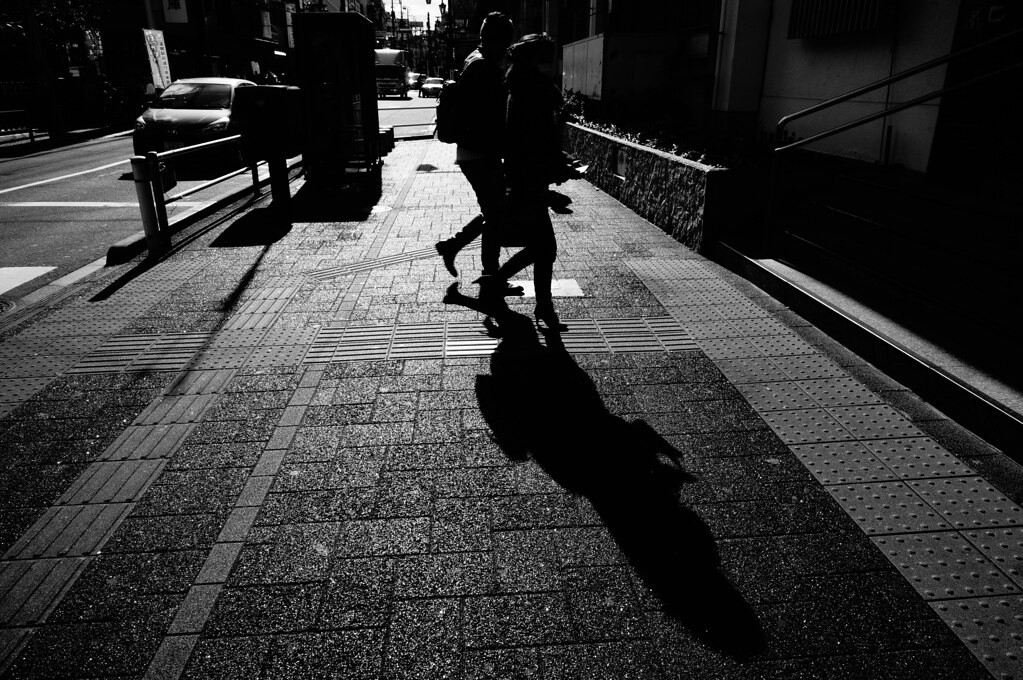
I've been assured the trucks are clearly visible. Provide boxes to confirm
[373,48,410,98]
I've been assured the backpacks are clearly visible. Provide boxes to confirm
[437,56,493,143]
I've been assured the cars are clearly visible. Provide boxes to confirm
[131,77,258,158]
[421,77,444,98]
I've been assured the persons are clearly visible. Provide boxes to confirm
[434,11,588,332]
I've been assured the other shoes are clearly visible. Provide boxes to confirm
[503,281,524,294]
[435,238,460,278]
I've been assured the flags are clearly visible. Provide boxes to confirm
[143,29,171,89]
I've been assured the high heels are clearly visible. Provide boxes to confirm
[533,303,568,329]
[472,275,500,317]
